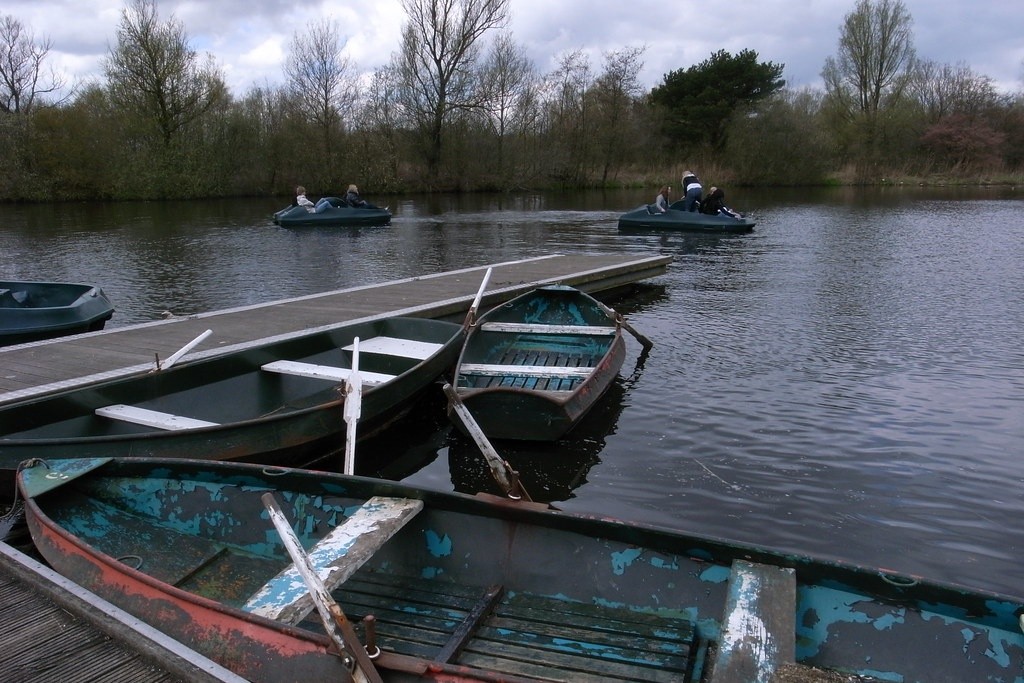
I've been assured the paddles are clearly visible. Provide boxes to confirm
[593,299,656,352]
[256,487,386,683]
[147,326,215,376]
[436,378,539,507]
[342,334,365,482]
[459,264,495,343]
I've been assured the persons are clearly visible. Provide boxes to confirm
[295,185,340,213]
[681,169,703,211]
[655,185,671,213]
[697,187,742,221]
[346,183,389,210]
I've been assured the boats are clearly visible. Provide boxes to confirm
[618,203,758,234]
[0,276,117,346]
[272,196,394,227]
[445,282,628,441]
[14,453,1024,683]
[0,317,470,483]
